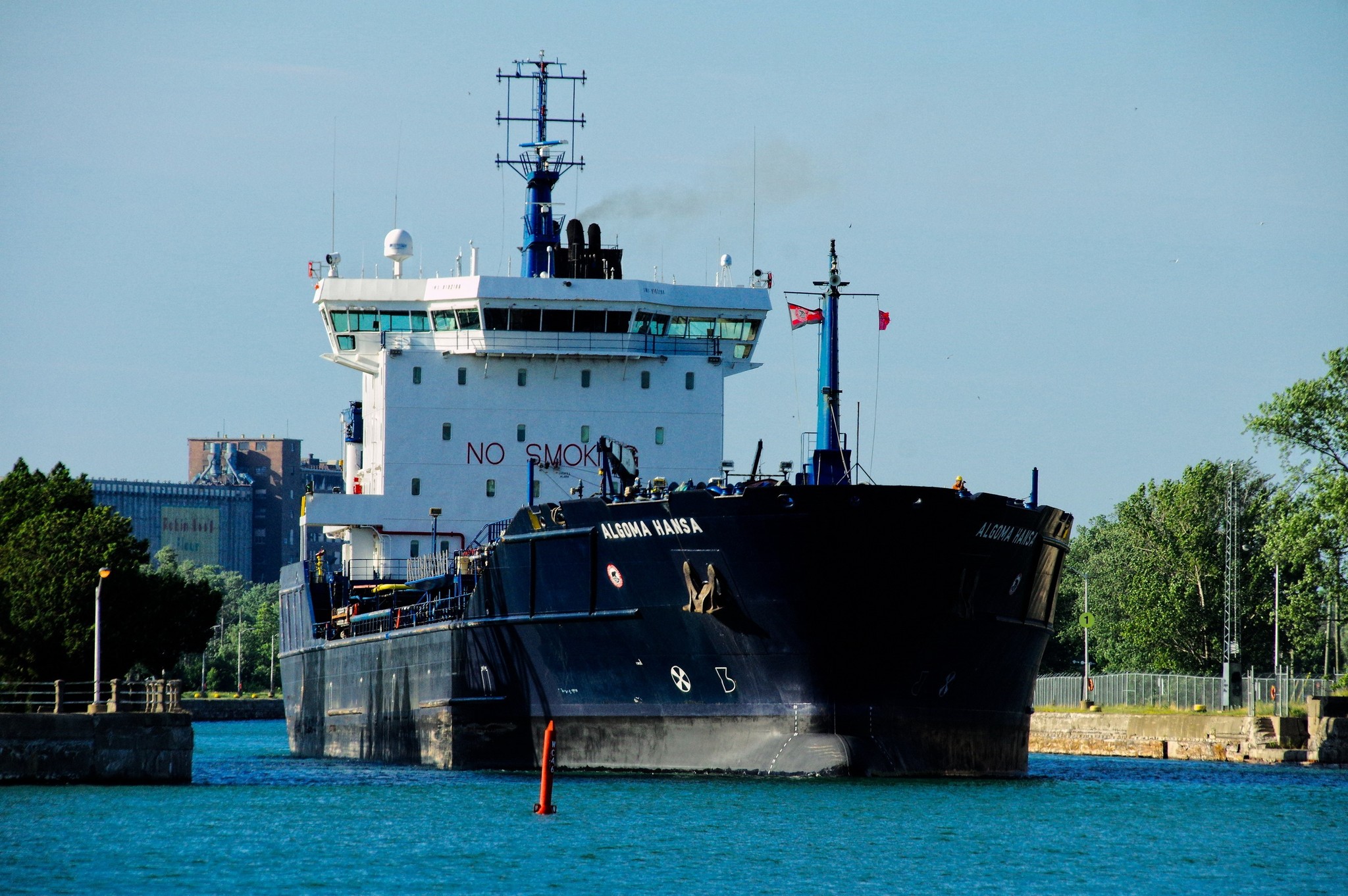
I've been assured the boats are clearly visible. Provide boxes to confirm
[276,47,1076,779]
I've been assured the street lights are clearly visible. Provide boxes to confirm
[269,633,279,697]
[1063,566,1089,701]
[428,507,443,577]
[237,627,257,696]
[201,624,221,693]
[1241,544,1279,674]
[94,567,111,705]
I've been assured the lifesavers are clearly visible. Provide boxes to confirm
[396,609,401,629]
[1088,679,1094,690]
[1271,685,1276,701]
[355,603,359,615]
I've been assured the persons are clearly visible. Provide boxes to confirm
[952,476,966,489]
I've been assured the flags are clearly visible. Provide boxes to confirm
[787,301,825,330]
[879,310,890,330]
